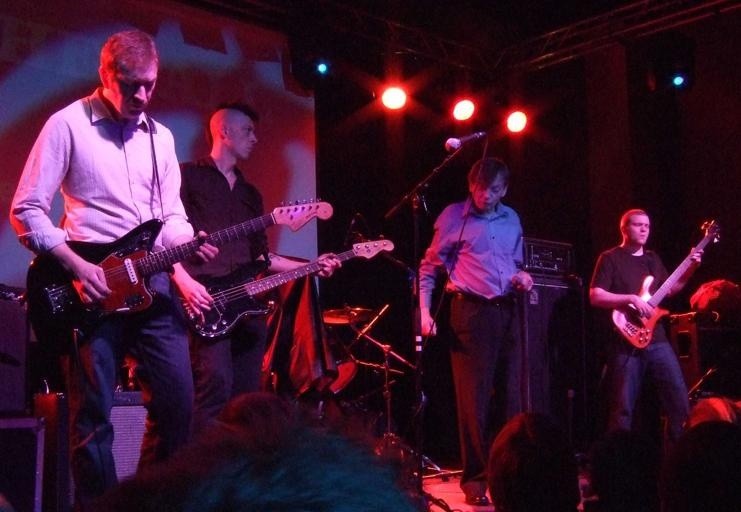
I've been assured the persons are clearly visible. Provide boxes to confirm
[588,208,705,456]
[413,157,534,506]
[179,101,342,443]
[486,396,741,512]
[116,393,421,512]
[9,29,221,497]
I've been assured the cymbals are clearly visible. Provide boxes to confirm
[324,308,375,323]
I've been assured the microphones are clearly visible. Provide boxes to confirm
[444,131,488,151]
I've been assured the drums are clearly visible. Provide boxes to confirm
[324,328,357,395]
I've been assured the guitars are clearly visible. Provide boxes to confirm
[612,220,720,348]
[177,234,393,343]
[26,197,334,356]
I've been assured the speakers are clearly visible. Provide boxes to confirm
[668,313,741,397]
[494,271,586,448]
